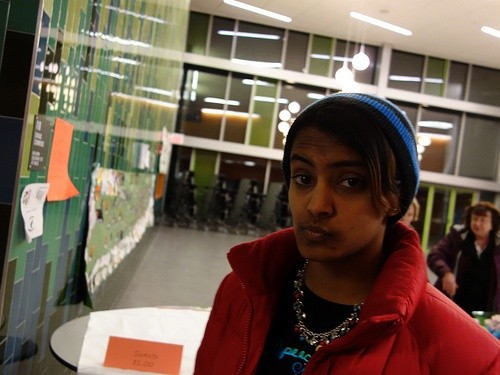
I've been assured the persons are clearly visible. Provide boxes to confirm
[399,193,421,227]
[426,202,500,317]
[192,92,500,375]
[167,169,291,236]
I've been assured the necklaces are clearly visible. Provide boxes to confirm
[293,256,369,347]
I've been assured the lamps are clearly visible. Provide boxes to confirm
[480,25,500,40]
[349,11,413,38]
[224,0,292,23]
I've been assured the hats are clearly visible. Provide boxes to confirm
[282,92,420,224]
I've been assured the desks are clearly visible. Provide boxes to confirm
[50,306,211,375]
